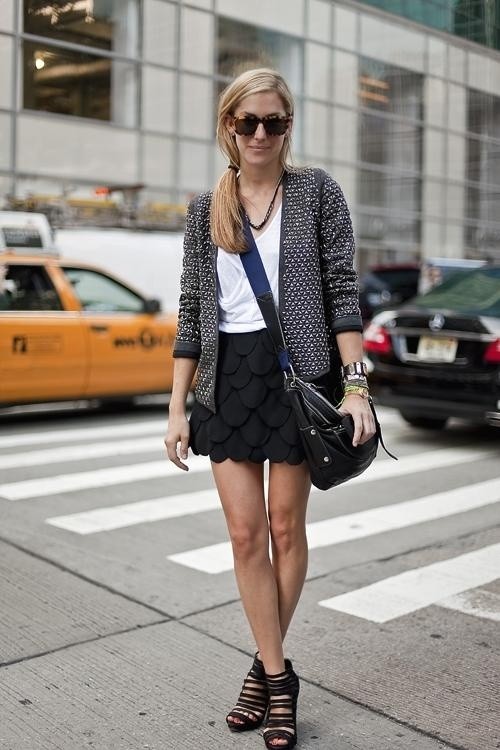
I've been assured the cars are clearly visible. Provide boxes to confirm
[0,251,197,406]
[359,255,499,435]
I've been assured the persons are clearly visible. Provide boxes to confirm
[164,66,378,749]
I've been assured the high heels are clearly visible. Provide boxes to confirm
[226,651,299,749]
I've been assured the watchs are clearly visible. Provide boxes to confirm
[343,385,369,398]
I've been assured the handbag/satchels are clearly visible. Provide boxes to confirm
[286,365,398,490]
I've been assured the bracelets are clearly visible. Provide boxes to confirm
[340,362,370,389]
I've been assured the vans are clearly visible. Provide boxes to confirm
[1,209,57,259]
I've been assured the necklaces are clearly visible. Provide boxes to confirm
[237,168,288,230]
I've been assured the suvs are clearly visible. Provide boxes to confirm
[355,258,491,329]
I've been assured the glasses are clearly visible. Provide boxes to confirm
[230,112,288,136]
